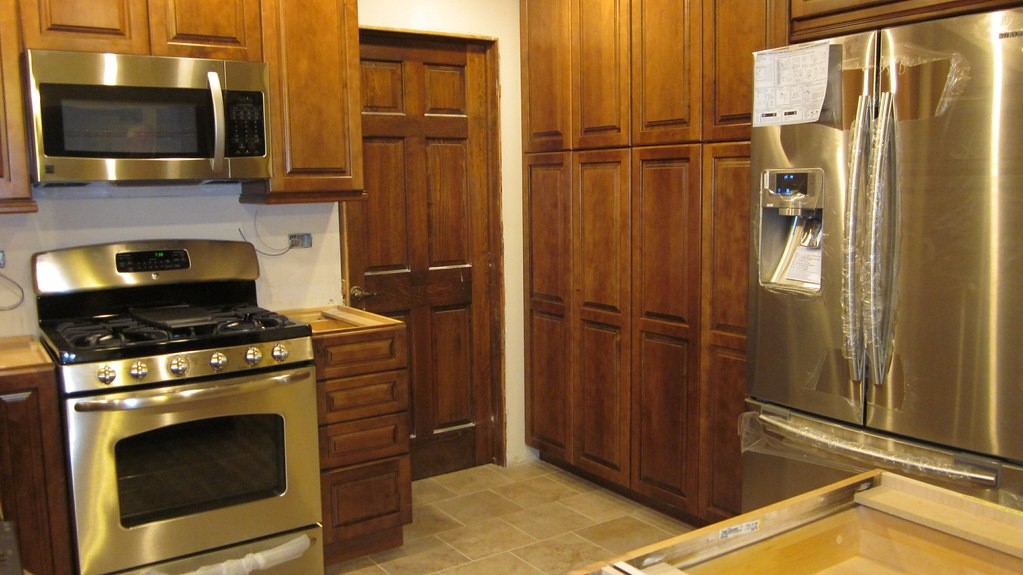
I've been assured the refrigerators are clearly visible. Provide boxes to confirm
[742,5,1023,534]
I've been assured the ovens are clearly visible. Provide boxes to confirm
[59,365,332,575]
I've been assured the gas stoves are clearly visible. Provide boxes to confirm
[29,238,315,393]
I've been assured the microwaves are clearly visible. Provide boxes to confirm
[21,45,273,186]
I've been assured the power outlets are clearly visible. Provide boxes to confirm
[288,233,313,249]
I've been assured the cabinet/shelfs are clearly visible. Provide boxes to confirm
[238,0,369,204]
[520,0,791,530]
[0,365,74,575]
[17,0,263,62]
[0,0,39,214]
[312,323,415,567]
